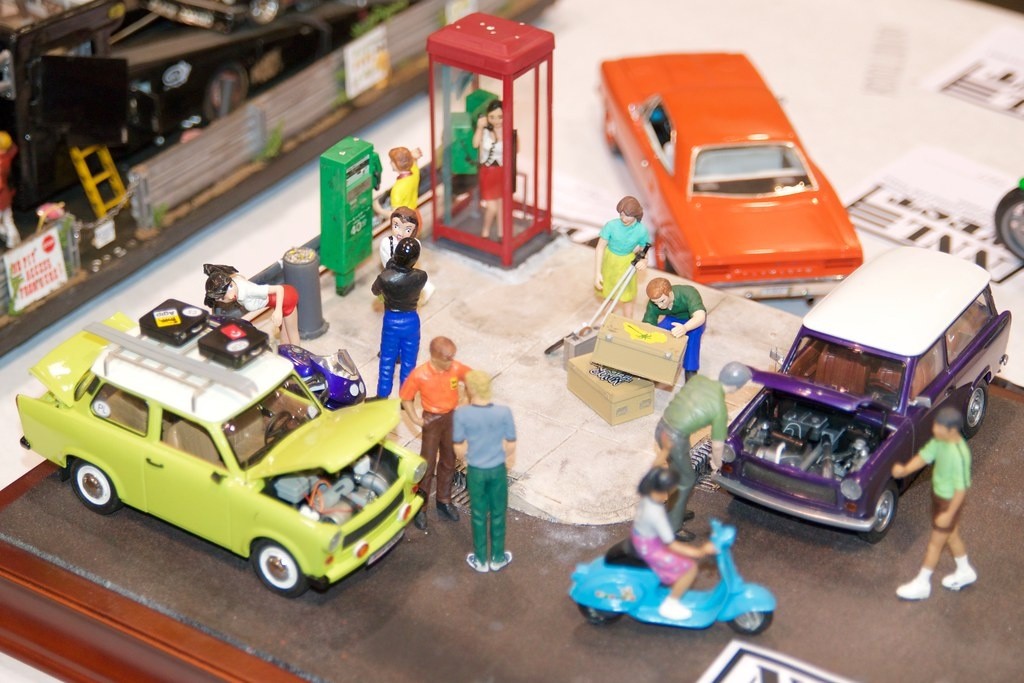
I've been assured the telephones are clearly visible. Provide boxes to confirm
[372,170,382,190]
[477,112,494,132]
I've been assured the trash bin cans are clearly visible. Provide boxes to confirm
[283,249,331,338]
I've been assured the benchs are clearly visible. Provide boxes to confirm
[170,403,267,464]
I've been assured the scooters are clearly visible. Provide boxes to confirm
[568,519,776,637]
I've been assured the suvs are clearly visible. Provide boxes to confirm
[706,242,1012,544]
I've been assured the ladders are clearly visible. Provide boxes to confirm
[71,138,130,222]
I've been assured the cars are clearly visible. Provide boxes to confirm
[597,52,864,306]
[14,309,429,599]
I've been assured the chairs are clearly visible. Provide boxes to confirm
[877,361,930,398]
[814,344,871,397]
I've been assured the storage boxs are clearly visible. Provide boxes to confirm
[591,312,689,386]
[566,352,655,427]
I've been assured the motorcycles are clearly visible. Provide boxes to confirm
[278,342,366,412]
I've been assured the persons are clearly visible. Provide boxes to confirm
[373,144,422,240]
[451,368,520,572]
[891,408,979,598]
[378,205,418,268]
[592,196,651,319]
[641,276,708,387]
[399,336,476,529]
[472,99,506,240]
[202,264,302,348]
[631,431,719,623]
[363,238,427,411]
[656,361,753,543]
